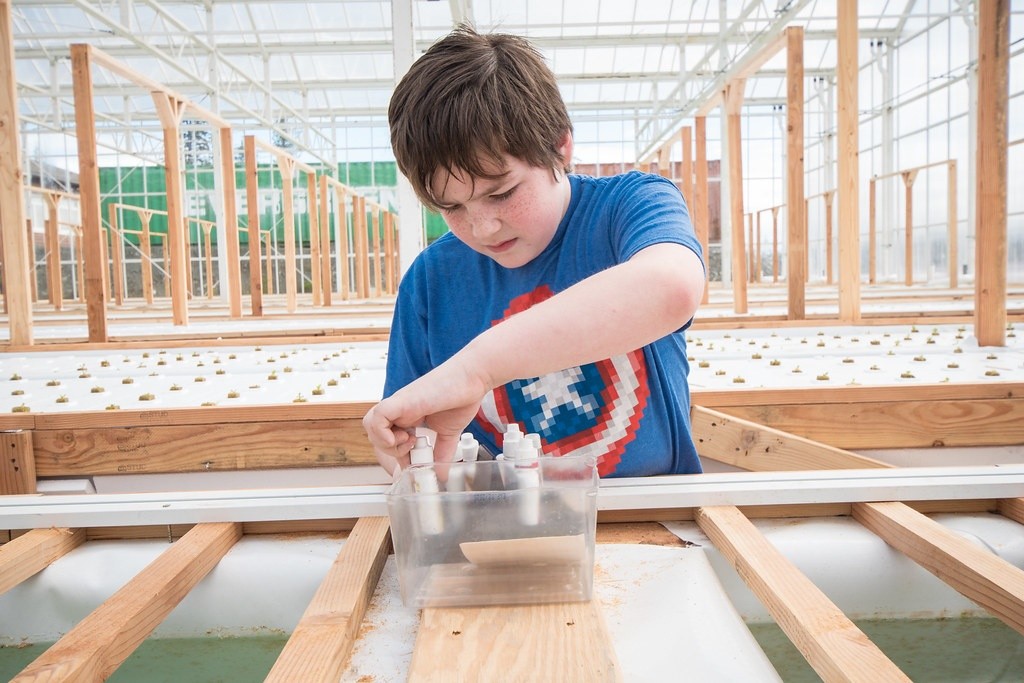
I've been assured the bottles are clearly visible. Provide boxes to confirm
[409,425,544,538]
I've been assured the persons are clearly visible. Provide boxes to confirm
[363,20,707,491]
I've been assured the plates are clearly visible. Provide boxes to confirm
[458,534,586,568]
